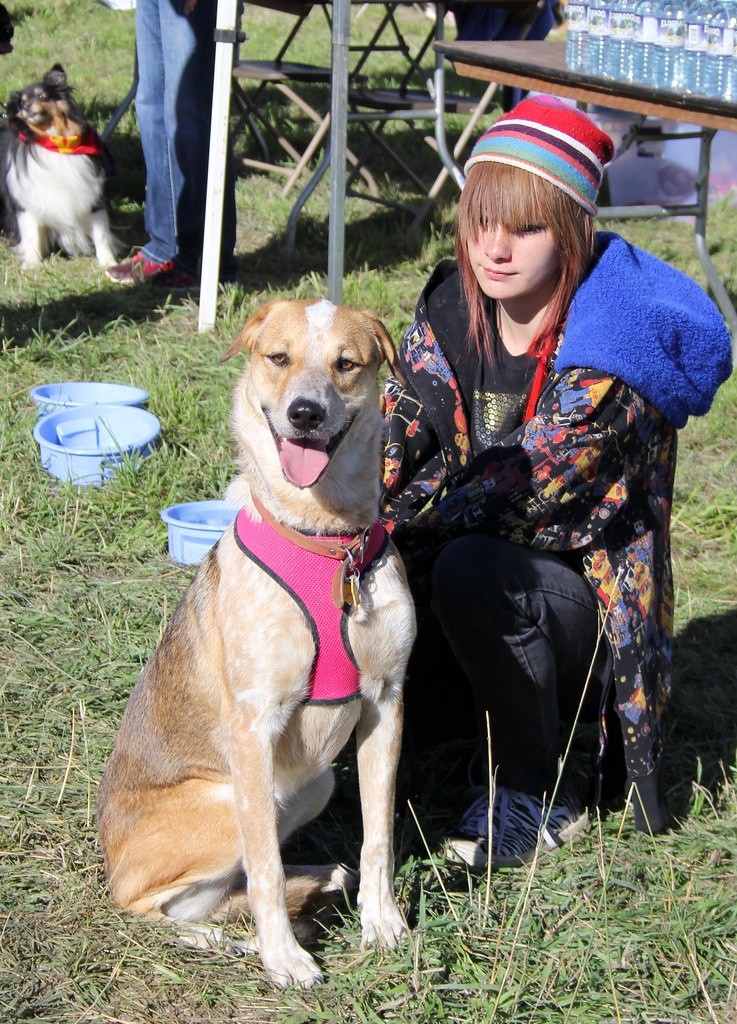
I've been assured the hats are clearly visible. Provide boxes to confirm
[462,92,614,217]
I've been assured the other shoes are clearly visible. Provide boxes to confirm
[105,252,198,291]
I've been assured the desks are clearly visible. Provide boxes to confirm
[431,37,737,340]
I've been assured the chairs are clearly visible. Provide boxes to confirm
[100,0,545,253]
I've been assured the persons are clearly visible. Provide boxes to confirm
[453,0,567,98]
[381,95,732,867]
[105,1,247,293]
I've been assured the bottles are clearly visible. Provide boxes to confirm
[563,0,737,105]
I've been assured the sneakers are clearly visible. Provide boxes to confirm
[444,775,590,872]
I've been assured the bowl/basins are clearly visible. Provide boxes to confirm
[161,500,242,566]
[33,405,160,488]
[30,380,149,419]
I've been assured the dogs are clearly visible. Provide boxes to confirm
[95,299,420,996]
[1,61,125,276]
